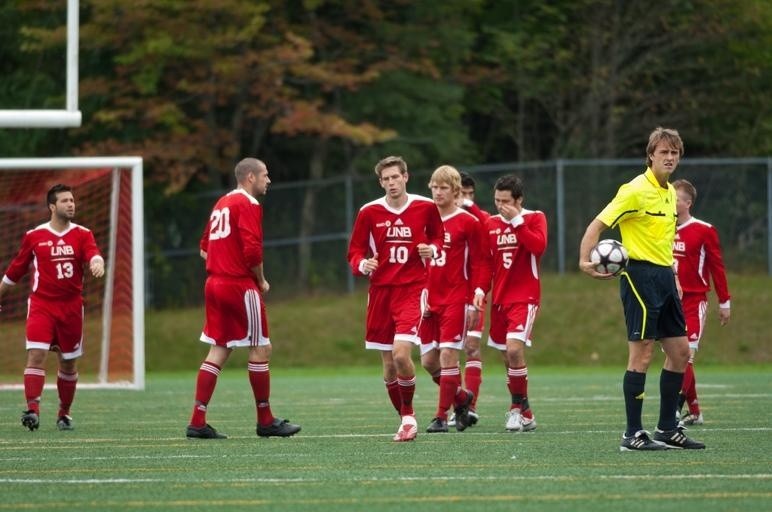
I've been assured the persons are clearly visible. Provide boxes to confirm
[472,177,548,433]
[458,172,492,427]
[671,179,732,426]
[346,154,444,442]
[0,184,105,430]
[186,153,301,437]
[580,128,711,452]
[420,165,483,432]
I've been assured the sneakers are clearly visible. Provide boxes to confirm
[678,412,704,425]
[256,418,300,437]
[652,426,705,449]
[57,413,73,431]
[619,430,666,452]
[448,389,479,431]
[20,410,40,431]
[506,409,522,431]
[518,414,537,432]
[391,411,450,442]
[186,424,227,440]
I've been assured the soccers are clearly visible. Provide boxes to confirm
[588,238,629,278]
